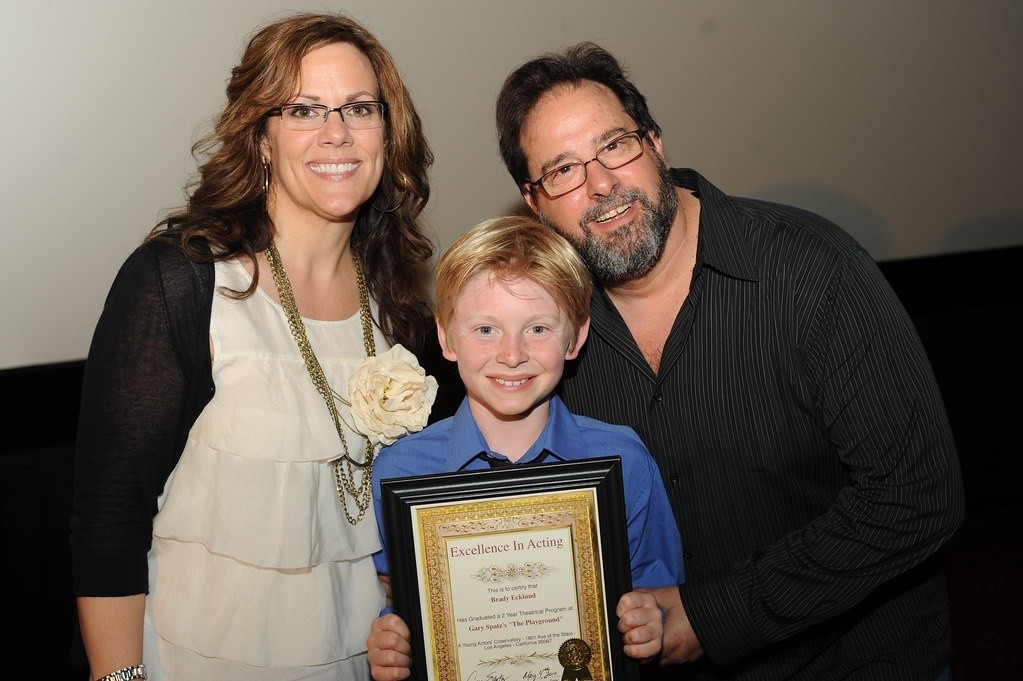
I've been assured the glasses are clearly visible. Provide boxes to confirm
[524,128,644,200]
[265,100,388,130]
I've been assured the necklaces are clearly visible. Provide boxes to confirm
[261,217,378,527]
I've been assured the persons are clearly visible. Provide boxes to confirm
[65,11,472,681]
[492,40,971,681]
[367,211,690,681]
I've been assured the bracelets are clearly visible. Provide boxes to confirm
[94,663,148,681]
